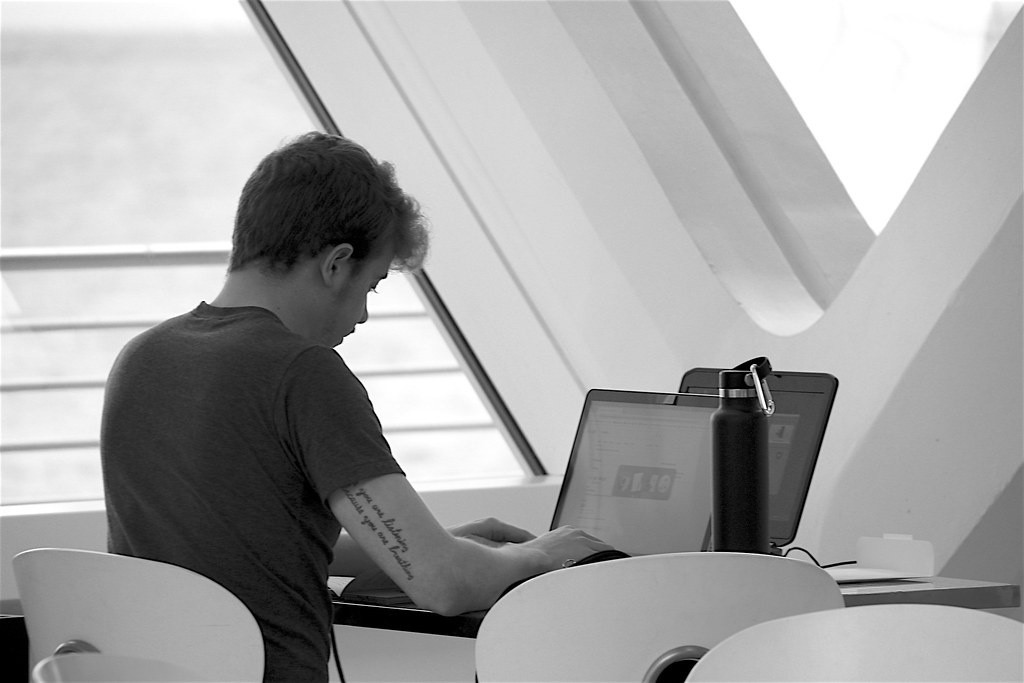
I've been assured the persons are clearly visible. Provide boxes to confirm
[99,132,621,683]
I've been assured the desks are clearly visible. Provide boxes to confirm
[323,561,1020,683]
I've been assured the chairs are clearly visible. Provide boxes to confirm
[684,604,1024,683]
[475,551,844,683]
[12,548,265,683]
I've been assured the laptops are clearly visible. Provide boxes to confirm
[549,368,836,558]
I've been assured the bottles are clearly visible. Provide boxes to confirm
[711,358,774,553]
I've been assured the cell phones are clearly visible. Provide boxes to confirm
[341,588,411,604]
